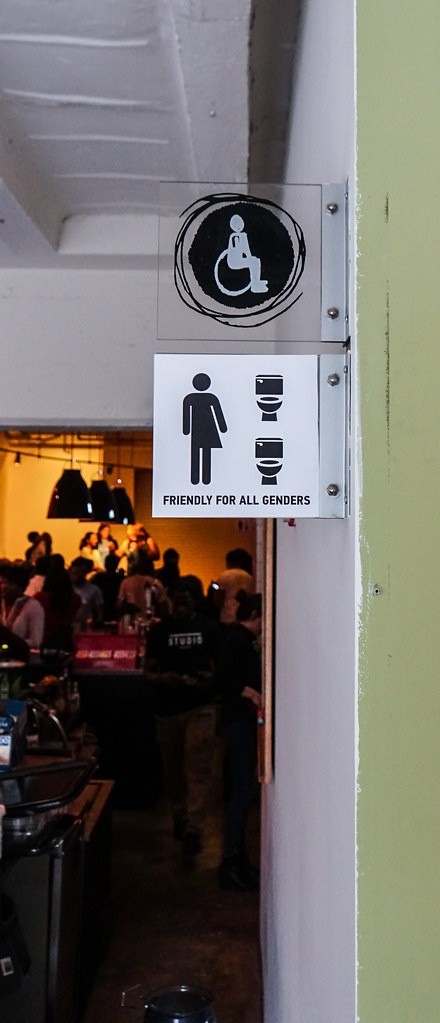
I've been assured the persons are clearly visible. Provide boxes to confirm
[0,524,261,892]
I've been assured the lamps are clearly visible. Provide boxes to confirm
[48,431,97,519]
[81,434,113,524]
[112,431,138,525]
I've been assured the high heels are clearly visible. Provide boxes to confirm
[218,856,259,891]
